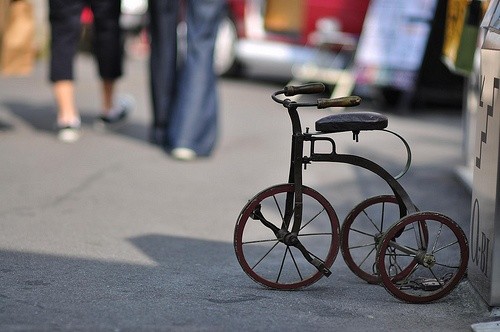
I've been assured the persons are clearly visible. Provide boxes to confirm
[47,1,138,142]
[146,1,230,161]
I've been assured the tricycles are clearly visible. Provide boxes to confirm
[234,83,469,304]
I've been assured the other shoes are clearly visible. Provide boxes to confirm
[172,146,196,158]
[98,95,130,127]
[55,116,82,142]
